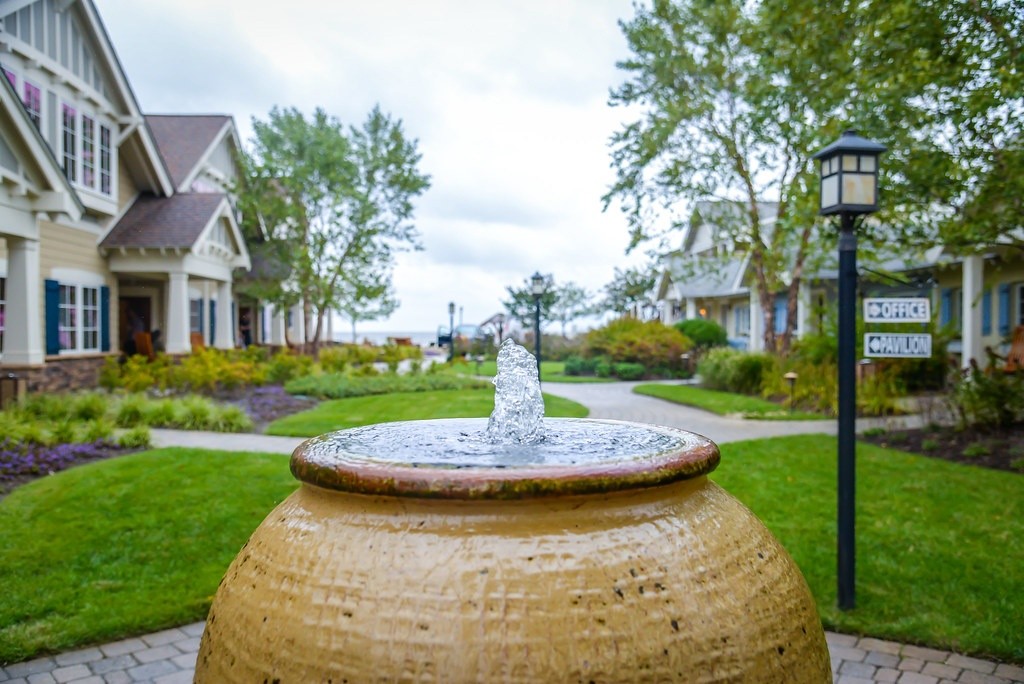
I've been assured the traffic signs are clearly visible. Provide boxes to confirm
[862,332,933,359]
[863,297,931,324]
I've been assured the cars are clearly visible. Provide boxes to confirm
[449,324,486,357]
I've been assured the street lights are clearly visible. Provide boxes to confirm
[812,130,890,611]
[530,272,545,389]
[448,302,456,360]
